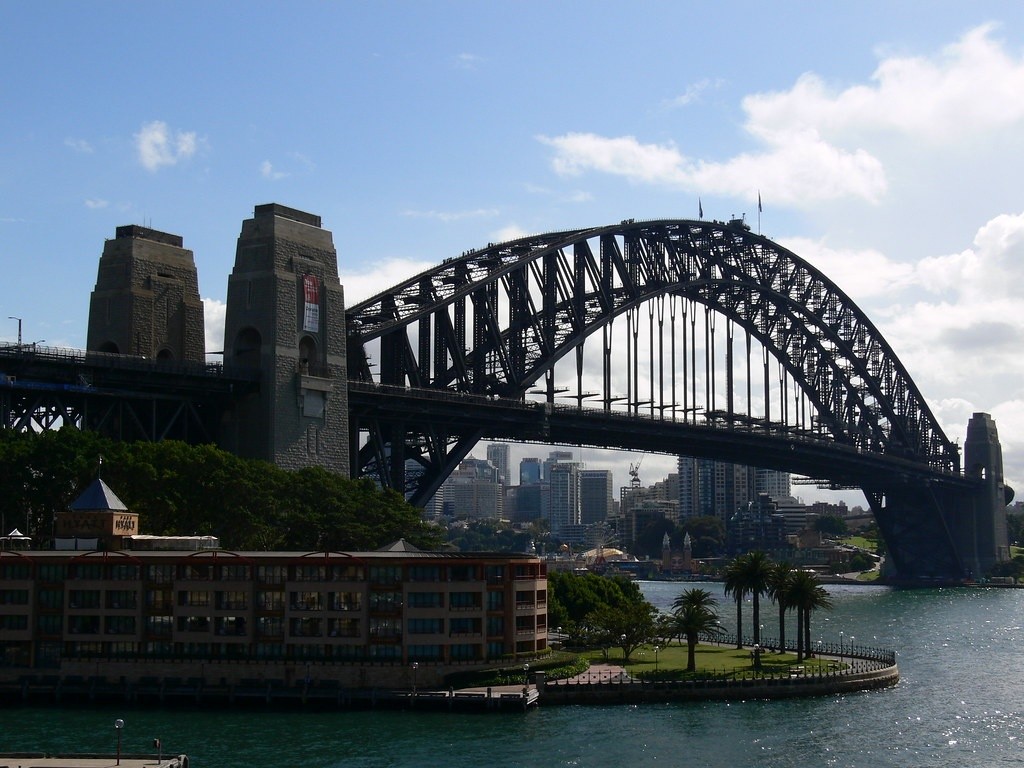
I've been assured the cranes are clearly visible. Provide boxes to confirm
[628,452,645,491]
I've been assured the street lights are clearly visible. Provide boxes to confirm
[7,315,23,354]
[816,641,823,676]
[838,631,844,663]
[653,645,660,683]
[556,626,563,653]
[759,624,764,648]
[33,340,45,352]
[605,630,611,663]
[412,661,419,696]
[114,719,125,765]
[850,635,855,667]
[620,633,627,667]
[523,662,530,695]
[715,620,721,646]
[753,643,759,678]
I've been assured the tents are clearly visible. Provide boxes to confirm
[0,528,31,551]
